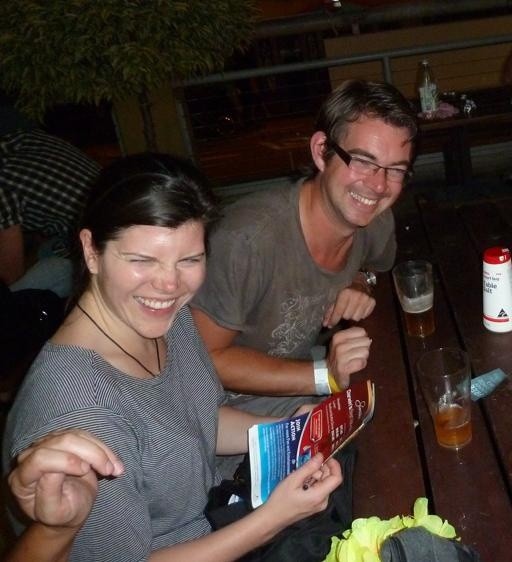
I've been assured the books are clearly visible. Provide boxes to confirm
[246,379,378,510]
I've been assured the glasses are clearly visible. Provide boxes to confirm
[325,138,415,185]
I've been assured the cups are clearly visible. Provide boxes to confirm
[416,348,472,450]
[392,259,438,338]
[483,248,512,334]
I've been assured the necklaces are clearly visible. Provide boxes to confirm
[74,303,161,382]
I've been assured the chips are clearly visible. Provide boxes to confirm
[321,496,456,561]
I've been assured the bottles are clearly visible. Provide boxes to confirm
[417,58,440,120]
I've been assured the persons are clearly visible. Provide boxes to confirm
[0,429,124,562]
[191,76,421,425]
[7,157,342,562]
[1,128,108,306]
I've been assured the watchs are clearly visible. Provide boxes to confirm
[358,266,376,289]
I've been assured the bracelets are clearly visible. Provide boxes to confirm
[313,358,331,399]
